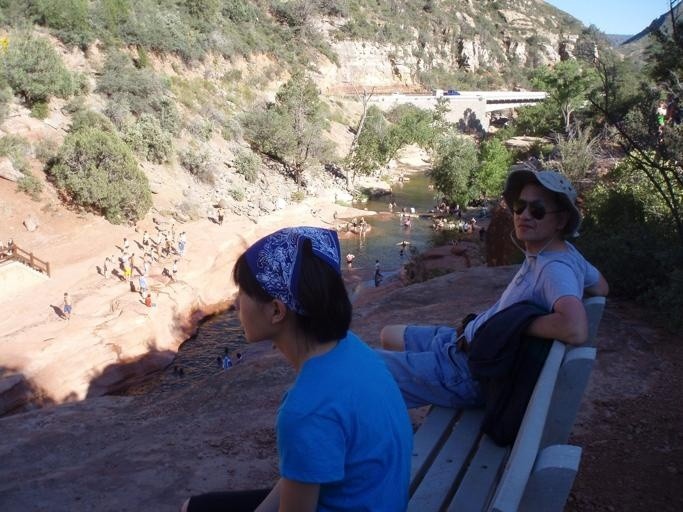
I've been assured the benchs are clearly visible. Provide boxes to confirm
[411,296,605,512]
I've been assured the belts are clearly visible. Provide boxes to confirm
[455,318,467,354]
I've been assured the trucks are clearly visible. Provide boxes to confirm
[443,90,459,94]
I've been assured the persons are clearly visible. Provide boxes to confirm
[174,365,178,372]
[371,164,611,411]
[222,352,232,371]
[375,259,383,279]
[59,292,72,322]
[346,251,355,272]
[216,356,222,363]
[102,220,187,307]
[352,200,477,257]
[178,368,184,375]
[180,225,414,512]
[235,353,242,365]
[217,207,224,225]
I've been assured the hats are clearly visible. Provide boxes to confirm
[501,169,583,241]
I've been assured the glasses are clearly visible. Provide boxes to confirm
[510,198,564,219]
